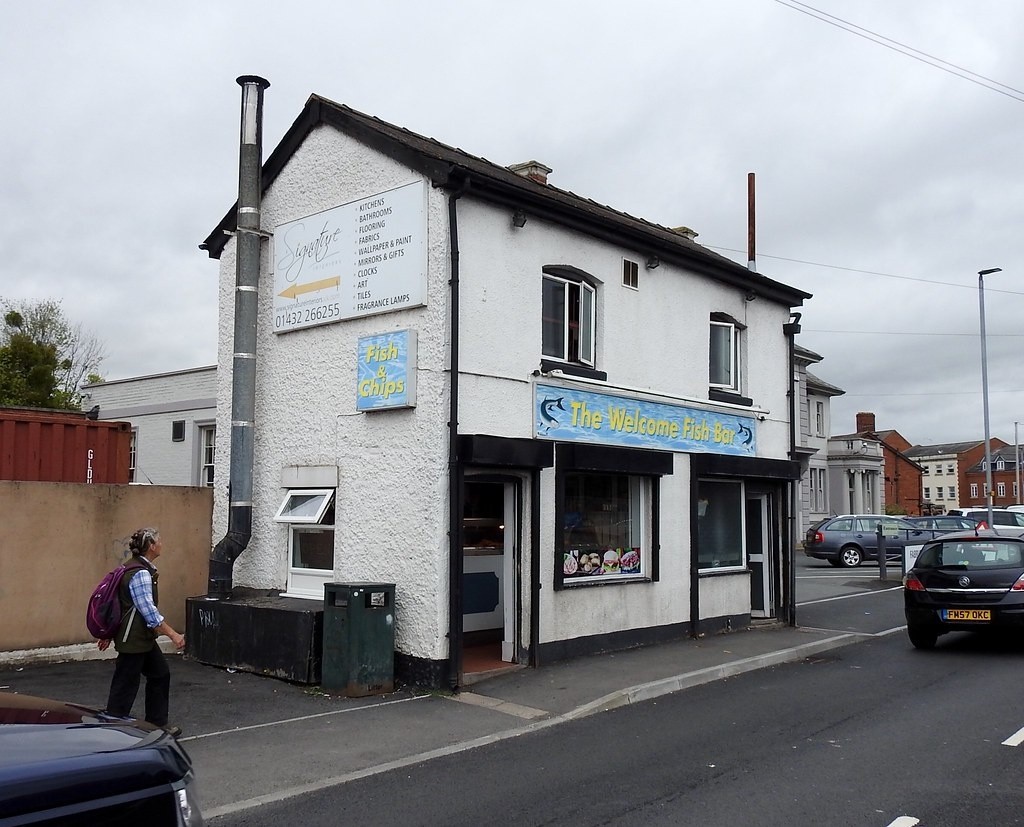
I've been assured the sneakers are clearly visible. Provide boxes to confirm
[163,724,183,737]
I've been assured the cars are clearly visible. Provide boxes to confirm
[893,515,999,538]
[904,507,1024,648]
[0,690,204,827]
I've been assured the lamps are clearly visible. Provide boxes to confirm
[648,255,659,269]
[512,209,526,227]
[978,268,1001,274]
[745,289,756,301]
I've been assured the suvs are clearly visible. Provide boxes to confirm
[803,510,945,567]
[937,508,1024,540]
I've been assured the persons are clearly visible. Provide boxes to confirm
[95,528,186,737]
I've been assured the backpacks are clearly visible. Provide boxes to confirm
[86,562,155,642]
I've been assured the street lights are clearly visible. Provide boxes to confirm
[977,267,1003,529]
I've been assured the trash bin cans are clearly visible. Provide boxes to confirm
[320,581,398,697]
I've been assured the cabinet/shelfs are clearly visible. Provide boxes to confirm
[463,518,504,645]
